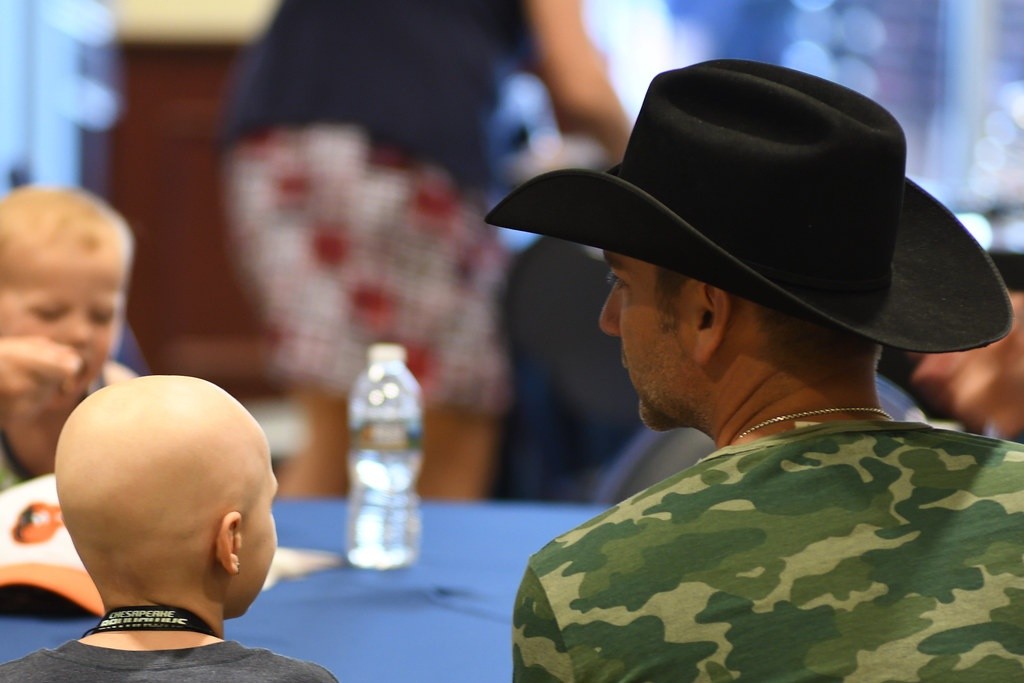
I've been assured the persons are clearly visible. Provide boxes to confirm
[0,375,338,683]
[219,0,634,507]
[486,61,1024,683]
[0,187,141,496]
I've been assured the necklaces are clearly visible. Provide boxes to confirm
[738,407,894,440]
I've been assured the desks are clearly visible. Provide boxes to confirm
[0,497,625,683]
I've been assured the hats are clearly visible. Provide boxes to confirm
[1,474,108,618]
[484,58,1013,353]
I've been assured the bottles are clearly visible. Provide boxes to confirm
[342,342,423,571]
